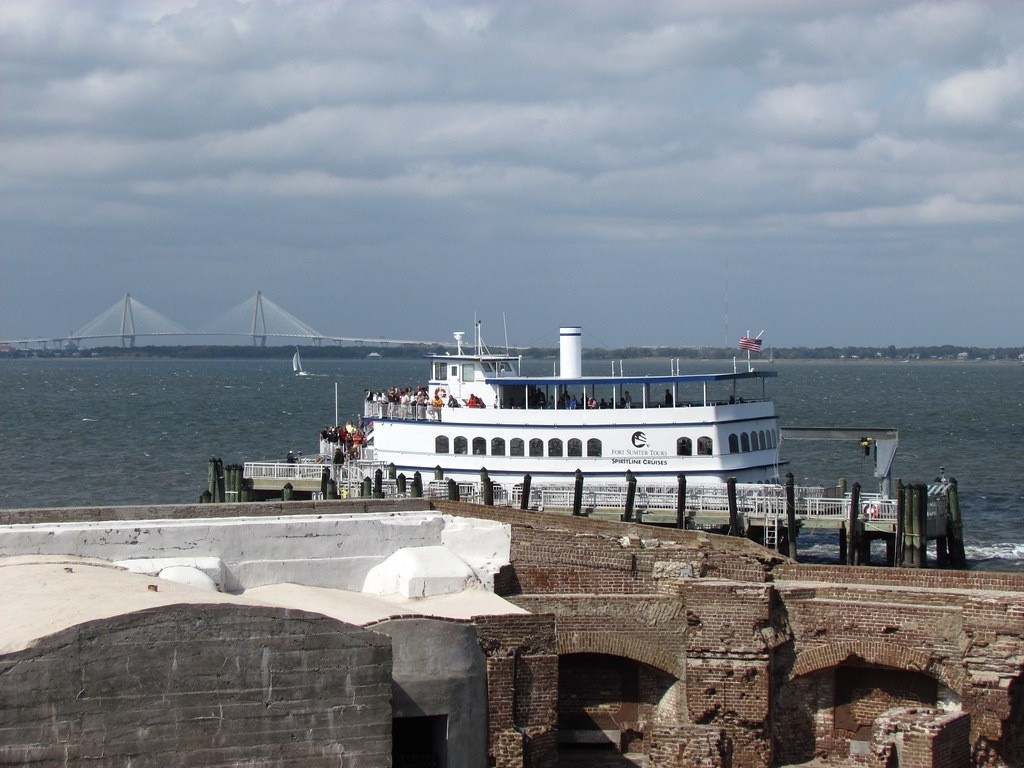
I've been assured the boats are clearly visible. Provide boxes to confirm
[243,307,951,552]
[367,352,382,360]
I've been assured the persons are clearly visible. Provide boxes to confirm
[625,391,631,409]
[599,398,607,409]
[448,395,458,407]
[494,395,502,408]
[665,389,673,407]
[466,394,485,408]
[320,414,367,453]
[609,398,617,409]
[508,398,516,406]
[581,393,589,405]
[285,450,298,476]
[588,397,598,409]
[729,395,735,405]
[432,396,442,418]
[333,446,346,478]
[365,385,428,419]
[557,392,577,409]
[620,398,625,405]
[529,389,554,408]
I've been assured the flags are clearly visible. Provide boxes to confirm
[738,338,762,352]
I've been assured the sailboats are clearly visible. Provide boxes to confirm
[292,346,317,377]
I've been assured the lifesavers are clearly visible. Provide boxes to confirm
[435,387,447,398]
[864,504,877,518]
